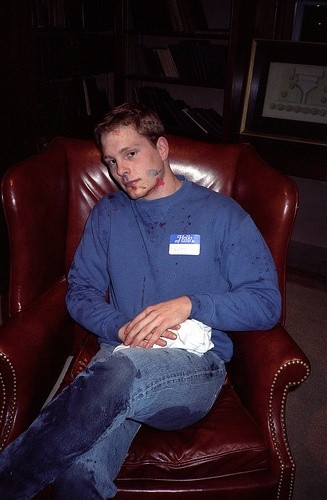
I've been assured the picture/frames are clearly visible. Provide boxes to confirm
[238,37,327,147]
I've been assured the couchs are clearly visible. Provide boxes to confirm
[0,135,312,500]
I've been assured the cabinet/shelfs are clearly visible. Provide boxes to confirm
[122,0,239,142]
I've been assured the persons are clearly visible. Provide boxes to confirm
[0,102,282,500]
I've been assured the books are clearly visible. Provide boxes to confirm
[132,86,223,138]
[142,40,228,84]
[166,0,198,32]
